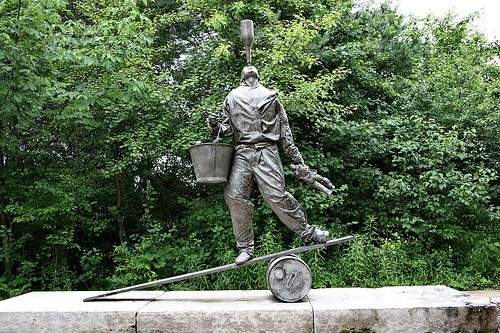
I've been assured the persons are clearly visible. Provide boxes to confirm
[289,163,335,196]
[207,65,329,265]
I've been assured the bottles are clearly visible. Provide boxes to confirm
[241,20,254,65]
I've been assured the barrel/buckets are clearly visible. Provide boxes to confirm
[266,254,312,303]
[188,123,233,183]
